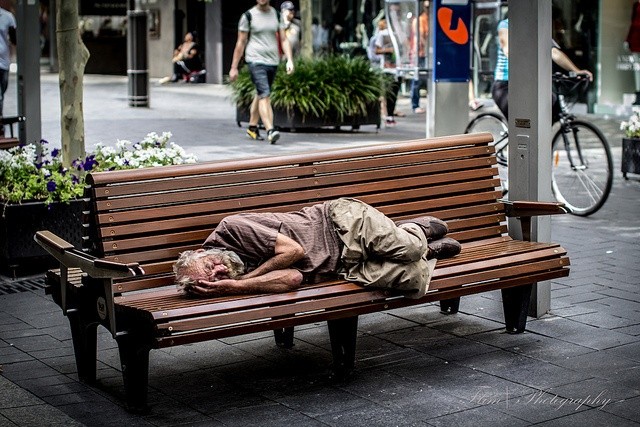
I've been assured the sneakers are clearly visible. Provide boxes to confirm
[267,129,280,144]
[394,216,449,242]
[471,100,484,111]
[414,108,425,113]
[246,128,264,140]
[383,116,399,127]
[393,111,406,117]
[427,237,461,260]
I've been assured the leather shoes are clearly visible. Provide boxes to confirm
[169,73,180,82]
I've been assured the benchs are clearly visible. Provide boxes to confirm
[33,131,573,416]
[1,115,26,150]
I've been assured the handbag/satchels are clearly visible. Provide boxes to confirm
[189,74,205,83]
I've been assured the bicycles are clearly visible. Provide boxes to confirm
[464,71,613,217]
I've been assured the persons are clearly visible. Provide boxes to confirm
[367,15,388,70]
[118,18,128,37]
[571,6,594,117]
[623,0,640,105]
[374,2,410,127]
[279,1,302,62]
[410,0,429,113]
[98,17,115,42]
[311,15,321,55]
[491,16,594,124]
[166,31,200,83]
[469,77,484,111]
[552,8,570,48]
[172,196,463,299]
[1,6,17,137]
[81,18,96,50]
[228,0,295,145]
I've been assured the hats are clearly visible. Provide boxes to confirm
[280,1,294,10]
